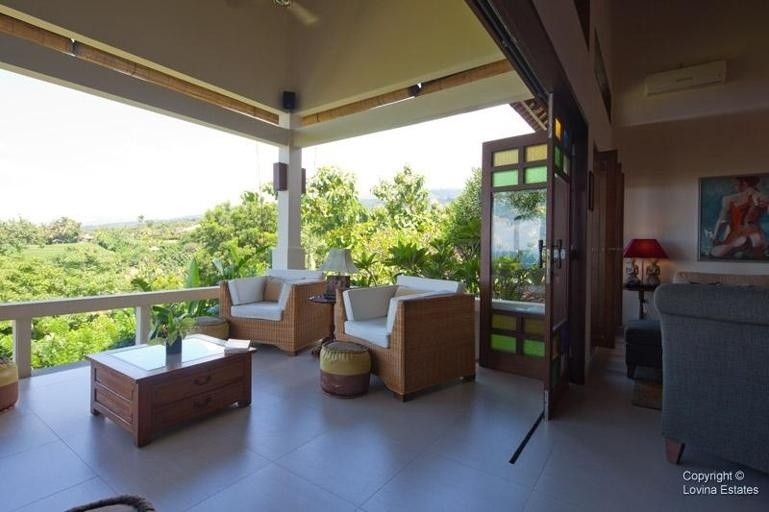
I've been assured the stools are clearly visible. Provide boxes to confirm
[320,340,371,399]
[193,317,229,339]
[625,321,662,378]
[0,367,18,412]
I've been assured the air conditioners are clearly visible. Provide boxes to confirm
[643,60,728,96]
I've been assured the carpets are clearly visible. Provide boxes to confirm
[631,364,662,410]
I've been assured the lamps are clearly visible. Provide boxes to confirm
[624,239,669,320]
[321,249,356,292]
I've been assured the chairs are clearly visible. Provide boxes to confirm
[219,269,335,356]
[334,275,475,403]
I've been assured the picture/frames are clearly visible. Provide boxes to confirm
[697,173,768,262]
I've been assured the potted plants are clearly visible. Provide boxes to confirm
[148,303,186,353]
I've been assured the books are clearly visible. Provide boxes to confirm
[223,338,251,352]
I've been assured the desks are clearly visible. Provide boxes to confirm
[308,295,335,358]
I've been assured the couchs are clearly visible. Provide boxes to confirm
[655,284,769,474]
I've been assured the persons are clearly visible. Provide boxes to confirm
[625,258,640,283]
[646,258,660,285]
[711,176,769,258]
